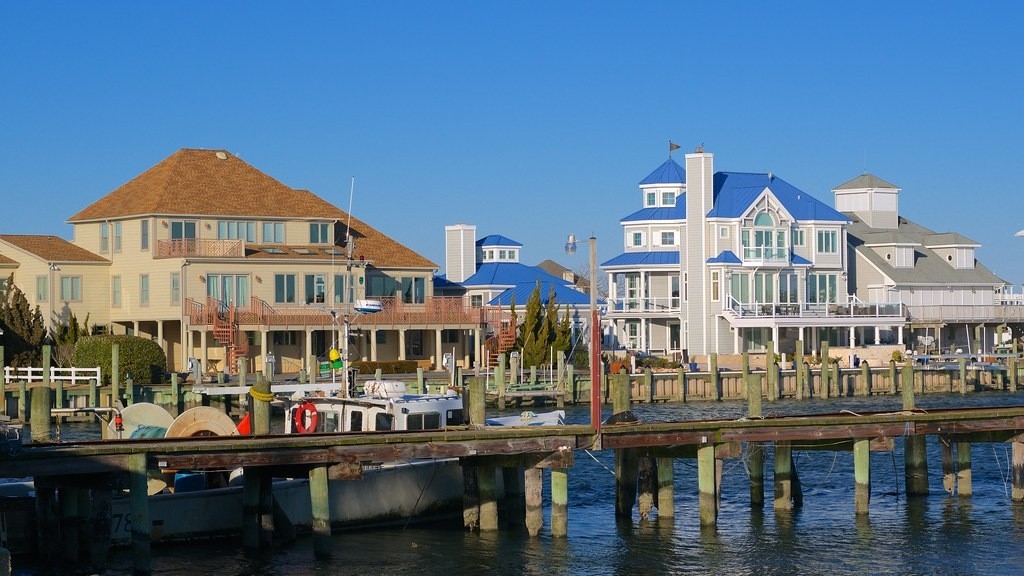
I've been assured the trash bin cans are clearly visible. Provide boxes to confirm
[848,352,860,369]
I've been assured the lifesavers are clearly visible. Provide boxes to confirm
[295,403,318,433]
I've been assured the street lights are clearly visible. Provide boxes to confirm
[565,235,601,426]
[318,306,350,398]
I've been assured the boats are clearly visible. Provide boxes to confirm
[0,232,565,555]
